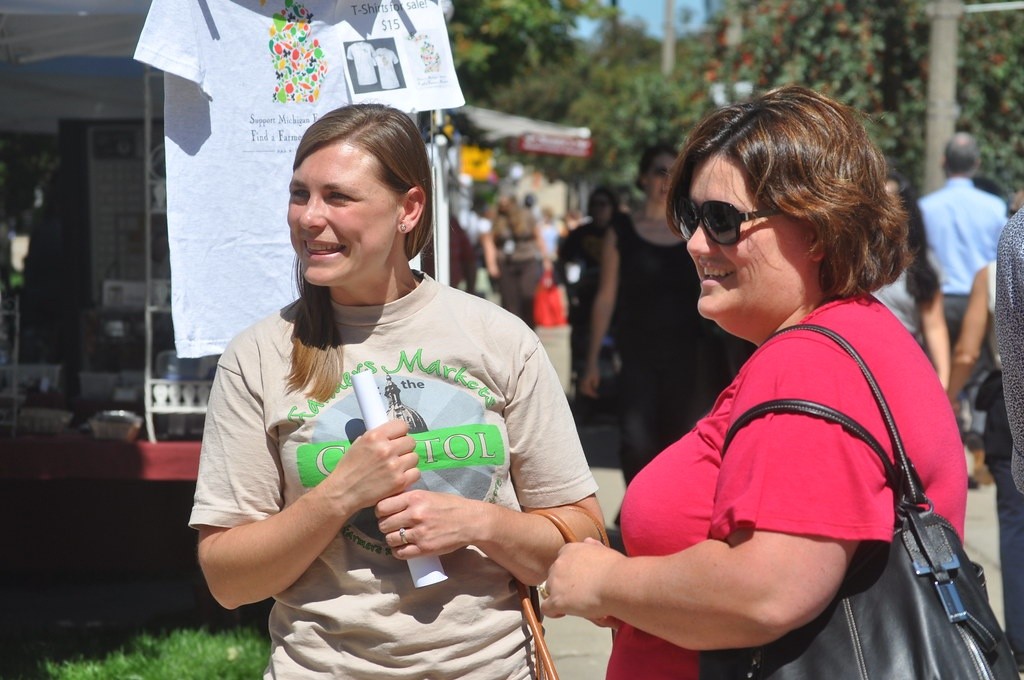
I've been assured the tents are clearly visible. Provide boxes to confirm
[0,2,593,468]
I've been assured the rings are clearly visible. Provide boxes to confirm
[534,580,549,598]
[399,527,409,544]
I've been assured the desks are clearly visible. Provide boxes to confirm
[0,396,241,628]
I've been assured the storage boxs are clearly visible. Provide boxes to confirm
[19,365,63,390]
[79,371,120,401]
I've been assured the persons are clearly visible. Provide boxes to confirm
[950,190,1024,672]
[911,128,1010,488]
[863,171,952,404]
[581,137,725,487]
[534,82,969,679]
[188,104,608,680]
[437,134,629,417]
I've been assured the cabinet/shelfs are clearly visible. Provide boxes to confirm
[0,294,21,437]
[140,65,213,446]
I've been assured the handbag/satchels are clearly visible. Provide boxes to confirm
[699,323,1019,680]
[535,270,568,328]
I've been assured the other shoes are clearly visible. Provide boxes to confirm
[963,452,978,488]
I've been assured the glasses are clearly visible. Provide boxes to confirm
[671,186,778,246]
[642,168,675,177]
